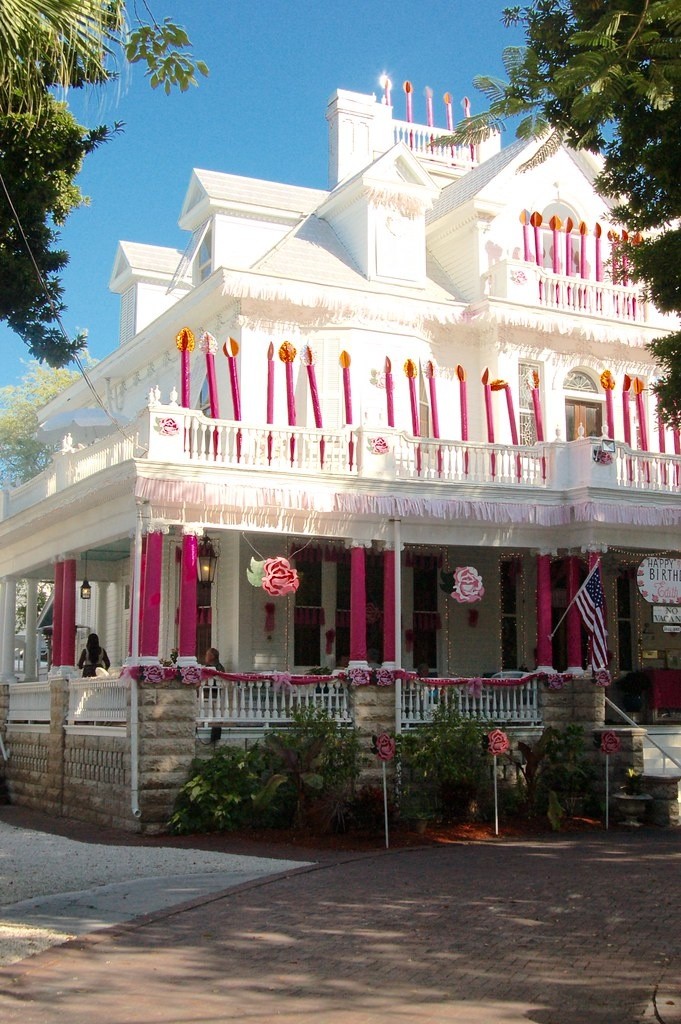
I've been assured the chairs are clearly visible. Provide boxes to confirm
[96,666,124,726]
[491,672,534,726]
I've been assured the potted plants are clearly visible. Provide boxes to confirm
[407,812,434,833]
[620,672,649,723]
[304,666,333,694]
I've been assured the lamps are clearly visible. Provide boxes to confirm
[196,530,219,587]
[592,438,615,452]
[80,551,92,600]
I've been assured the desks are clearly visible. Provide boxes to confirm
[627,669,681,724]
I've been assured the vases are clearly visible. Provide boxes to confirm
[613,793,653,827]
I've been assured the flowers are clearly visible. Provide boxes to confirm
[621,769,643,796]
[158,648,181,672]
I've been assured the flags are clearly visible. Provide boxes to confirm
[576,568,609,673]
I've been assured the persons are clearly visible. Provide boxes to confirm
[78,633,110,677]
[203,648,225,699]
[416,663,429,678]
[367,648,382,668]
